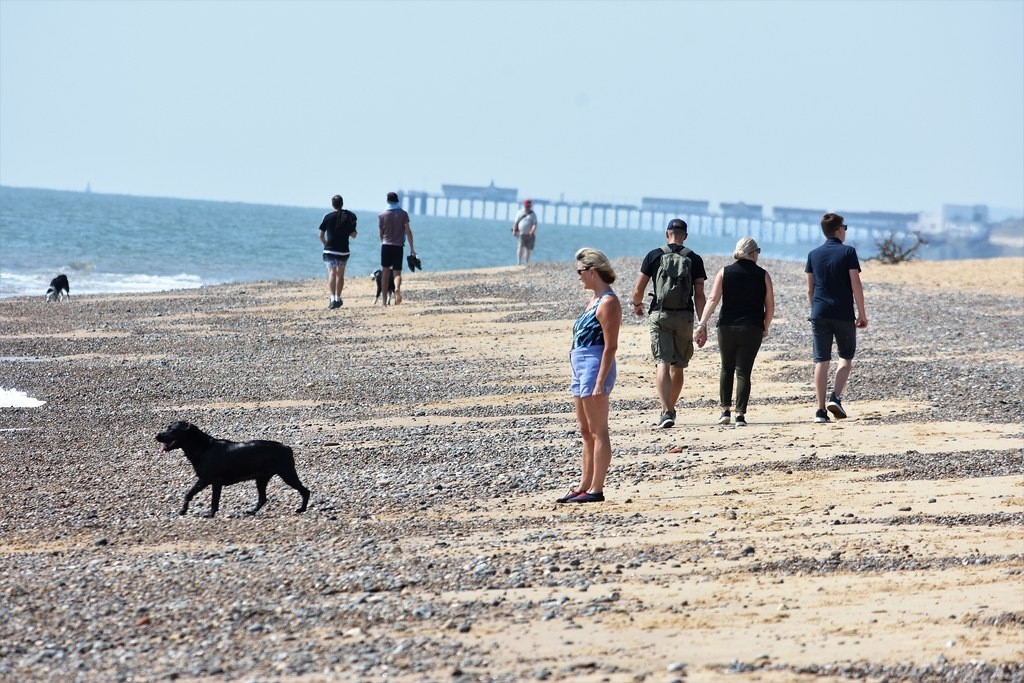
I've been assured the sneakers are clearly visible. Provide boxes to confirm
[671,411,676,420]
[814,409,830,423]
[736,416,747,426]
[658,411,674,427]
[718,410,730,424]
[827,392,847,418]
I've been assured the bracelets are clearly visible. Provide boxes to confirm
[697,322,706,327]
[632,301,642,307]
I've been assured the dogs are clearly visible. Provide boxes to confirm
[371,269,396,306]
[46,275,71,304]
[153,420,310,519]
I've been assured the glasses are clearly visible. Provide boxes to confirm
[577,266,591,275]
[836,225,847,231]
[749,248,760,254]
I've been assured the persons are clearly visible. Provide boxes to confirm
[556,247,622,504]
[513,199,537,266]
[378,192,416,306]
[694,238,775,426]
[805,212,868,422]
[634,218,708,425]
[319,195,357,309]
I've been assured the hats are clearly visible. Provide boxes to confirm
[667,219,687,233]
[524,200,530,205]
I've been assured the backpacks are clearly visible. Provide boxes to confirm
[655,245,692,308]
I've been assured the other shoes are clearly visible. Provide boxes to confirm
[407,255,414,272]
[556,491,580,502]
[569,492,604,503]
[414,257,422,270]
[328,300,335,309]
[335,298,343,307]
